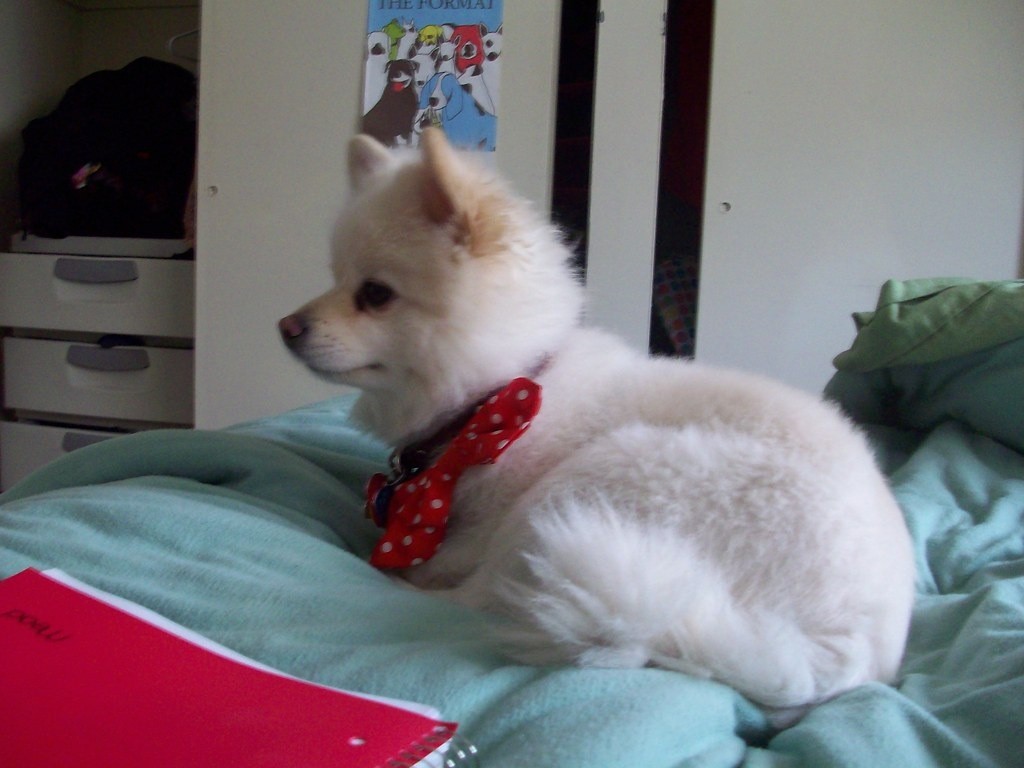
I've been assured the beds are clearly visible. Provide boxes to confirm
[2,279,1024,768]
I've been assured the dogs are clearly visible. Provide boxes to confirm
[278,122,915,732]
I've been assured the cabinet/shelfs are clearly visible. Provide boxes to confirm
[0,233,199,491]
